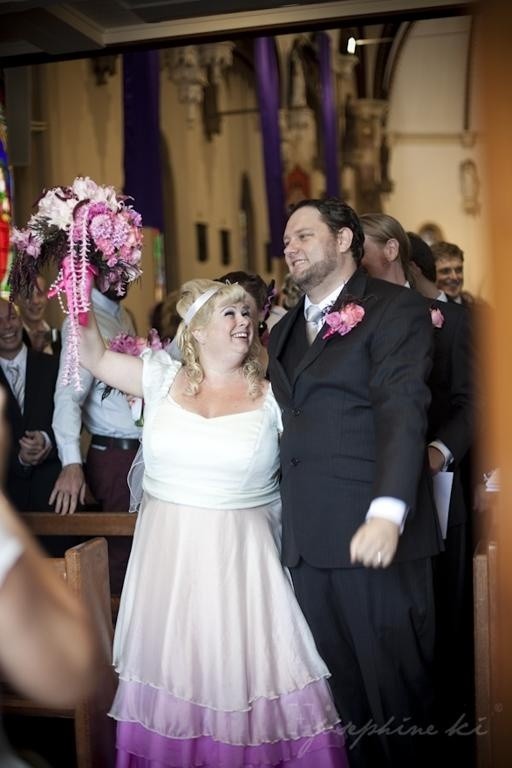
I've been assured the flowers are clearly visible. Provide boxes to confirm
[5,177,144,320]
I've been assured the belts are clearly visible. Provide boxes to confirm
[90,433,139,449]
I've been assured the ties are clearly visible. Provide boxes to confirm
[305,307,324,346]
[7,363,25,414]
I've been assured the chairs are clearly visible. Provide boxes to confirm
[0,536,127,767]
